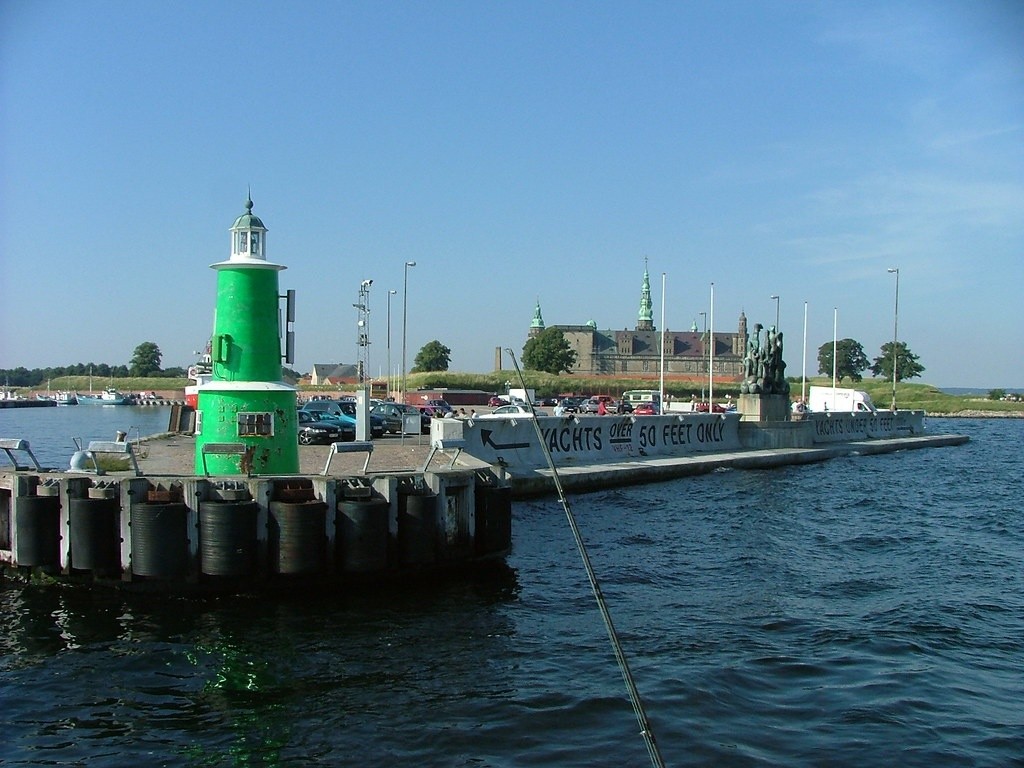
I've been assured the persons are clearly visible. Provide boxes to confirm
[617,399,629,414]
[666,397,695,412]
[791,399,808,413]
[433,408,478,418]
[598,398,613,416]
[553,399,565,416]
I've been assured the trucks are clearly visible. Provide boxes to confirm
[806,385,878,412]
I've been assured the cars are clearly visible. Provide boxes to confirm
[297,395,457,447]
[696,402,727,413]
[478,389,664,419]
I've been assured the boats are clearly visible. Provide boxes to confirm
[75,387,128,405]
[37,378,78,406]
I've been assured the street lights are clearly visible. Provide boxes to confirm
[886,267,901,409]
[386,289,398,405]
[403,261,417,404]
[699,311,707,402]
[769,294,780,333]
